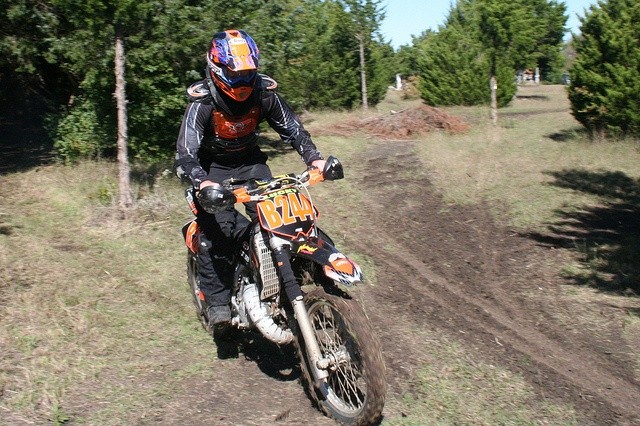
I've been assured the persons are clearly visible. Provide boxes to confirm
[174,30,328,332]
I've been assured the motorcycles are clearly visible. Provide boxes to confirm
[183,170,386,426]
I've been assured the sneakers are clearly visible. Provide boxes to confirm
[208,305,232,325]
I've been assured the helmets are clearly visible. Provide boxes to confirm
[207,29,260,102]
